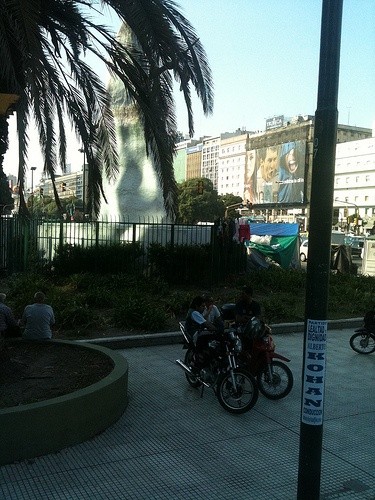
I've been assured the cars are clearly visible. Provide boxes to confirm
[300,239,308,262]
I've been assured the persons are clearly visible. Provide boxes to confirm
[233,213,245,239]
[245,145,304,205]
[183,287,264,338]
[0,292,55,339]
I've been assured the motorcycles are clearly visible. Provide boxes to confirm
[350,308,375,354]
[175,319,259,414]
[221,303,294,400]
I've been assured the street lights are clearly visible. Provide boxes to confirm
[31,167,37,218]
[78,149,86,221]
